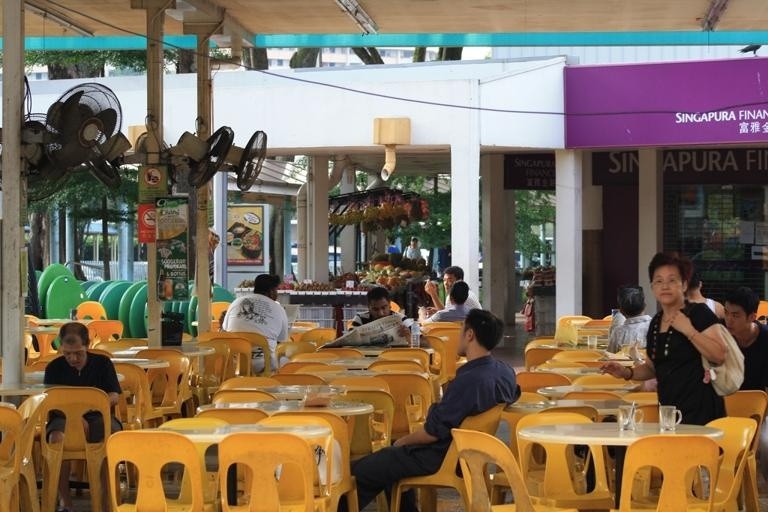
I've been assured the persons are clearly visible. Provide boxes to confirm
[222,275,290,370]
[419,281,470,325]
[387,237,400,257]
[606,284,653,352]
[600,251,728,495]
[424,267,482,314]
[348,287,411,347]
[609,312,626,342]
[339,309,522,512]
[686,273,725,321]
[44,322,122,512]
[721,287,767,391]
[404,237,421,260]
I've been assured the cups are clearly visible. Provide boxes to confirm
[70,309,78,320]
[611,308,620,319]
[329,384,348,408]
[164,279,173,299]
[586,335,597,351]
[621,343,634,359]
[618,404,645,433]
[658,405,683,433]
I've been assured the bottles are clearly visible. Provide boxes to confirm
[410,318,421,350]
[157,268,165,298]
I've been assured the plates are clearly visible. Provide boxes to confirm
[230,238,243,249]
[226,231,234,243]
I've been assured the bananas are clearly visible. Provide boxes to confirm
[328,202,403,226]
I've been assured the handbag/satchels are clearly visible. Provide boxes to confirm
[700,324,745,396]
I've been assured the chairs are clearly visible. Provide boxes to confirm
[1,300,768,512]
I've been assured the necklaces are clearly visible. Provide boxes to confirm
[662,315,672,323]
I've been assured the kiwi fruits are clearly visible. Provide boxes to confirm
[293,282,336,292]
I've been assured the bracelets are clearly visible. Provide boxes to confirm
[688,332,696,342]
[625,367,634,380]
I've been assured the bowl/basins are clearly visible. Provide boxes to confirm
[240,229,263,254]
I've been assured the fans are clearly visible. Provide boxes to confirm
[0,82,161,204]
[162,125,235,189]
[216,131,268,192]
